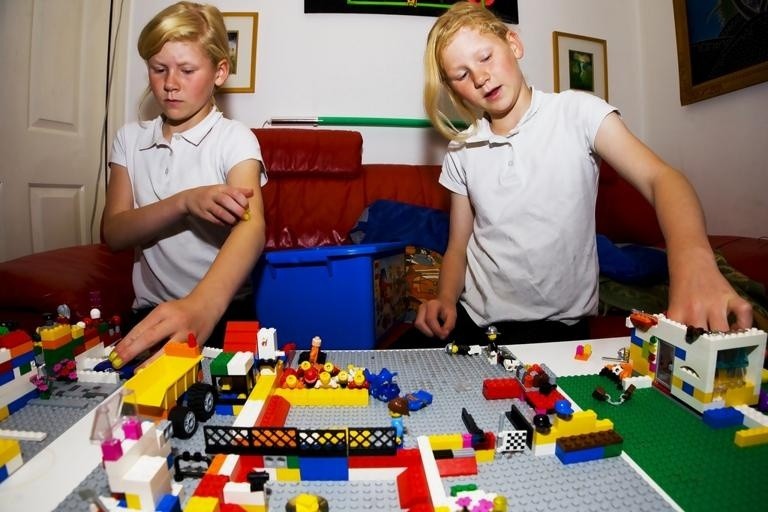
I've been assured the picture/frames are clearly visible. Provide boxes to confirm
[671,0,768,106]
[209,11,259,93]
[303,1,520,27]
[552,31,608,106]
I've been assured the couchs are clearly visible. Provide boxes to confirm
[6,127,766,376]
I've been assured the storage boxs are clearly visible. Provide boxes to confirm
[253,240,407,354]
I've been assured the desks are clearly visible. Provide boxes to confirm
[0,326,768,512]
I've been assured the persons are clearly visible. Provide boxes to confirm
[100,0,269,375]
[389,2,755,348]
